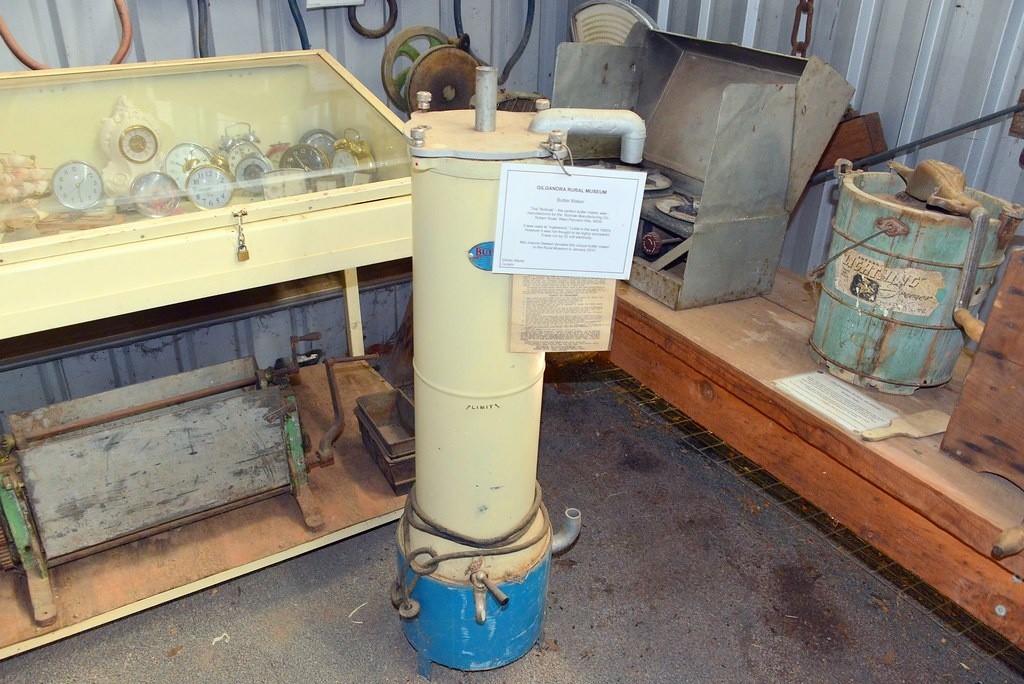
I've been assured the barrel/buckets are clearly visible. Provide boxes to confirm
[808,157,1024,395]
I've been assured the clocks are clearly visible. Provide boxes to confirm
[99,99,161,202]
[298,128,337,162]
[226,123,262,175]
[162,141,212,195]
[52,160,104,217]
[185,145,232,212]
[233,152,273,192]
[134,172,180,218]
[280,144,329,189]
[331,128,376,185]
